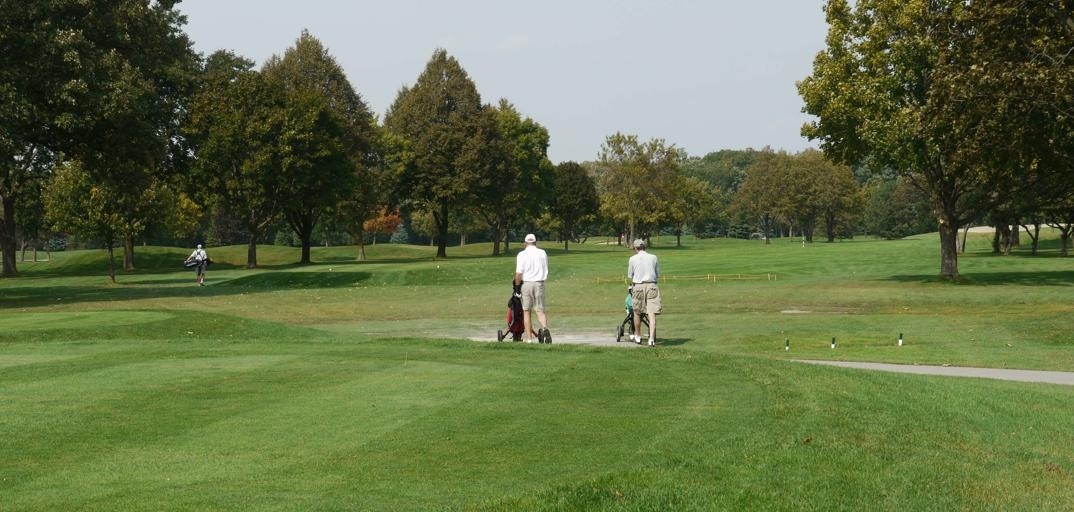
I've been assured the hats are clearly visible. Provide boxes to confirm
[525,234,536,243]
[632,239,646,249]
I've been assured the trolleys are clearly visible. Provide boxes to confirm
[498,281,543,344]
[618,281,657,339]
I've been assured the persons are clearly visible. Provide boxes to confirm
[514,234,552,343]
[185,244,208,286]
[627,239,661,347]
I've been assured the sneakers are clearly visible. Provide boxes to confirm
[648,340,655,346]
[523,339,532,343]
[543,327,552,343]
[629,334,642,344]
